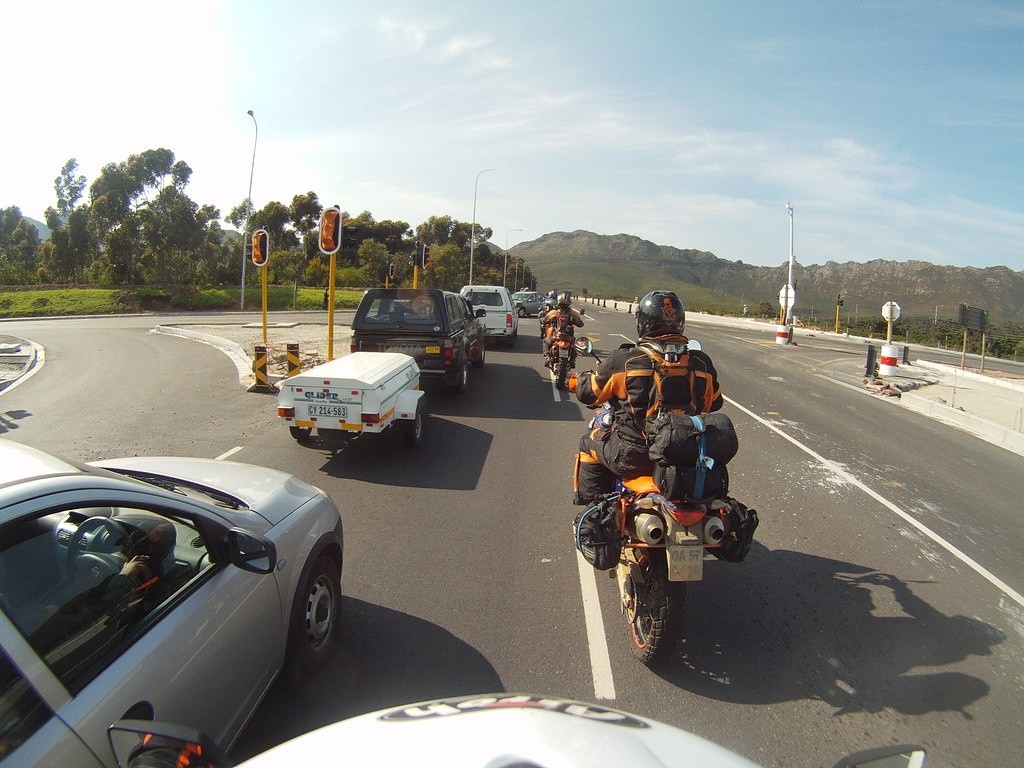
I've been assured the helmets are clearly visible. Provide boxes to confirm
[545,290,557,299]
[557,293,572,306]
[635,290,684,339]
[234,690,757,768]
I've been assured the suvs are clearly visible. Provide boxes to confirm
[351,286,487,396]
[457,285,522,347]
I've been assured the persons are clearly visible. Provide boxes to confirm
[522,287,530,292]
[538,290,584,366]
[741,304,748,317]
[570,290,724,506]
[0,522,149,655]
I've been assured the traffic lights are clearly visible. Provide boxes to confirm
[343,225,357,249]
[390,264,395,279]
[318,207,341,255]
[252,228,268,267]
[839,299,844,307]
[421,244,430,270]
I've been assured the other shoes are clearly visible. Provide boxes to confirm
[571,357,575,368]
[544,357,553,366]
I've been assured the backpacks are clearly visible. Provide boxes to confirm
[637,343,697,420]
[557,309,570,328]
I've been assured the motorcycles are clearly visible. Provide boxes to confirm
[536,308,548,339]
[539,308,585,391]
[565,336,760,667]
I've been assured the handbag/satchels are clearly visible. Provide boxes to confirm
[649,413,739,501]
[704,497,759,562]
[573,494,621,570]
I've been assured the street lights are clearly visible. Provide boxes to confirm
[503,228,522,287]
[469,167,495,285]
[522,262,540,289]
[241,110,258,310]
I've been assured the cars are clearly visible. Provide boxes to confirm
[511,292,545,318]
[1,434,342,768]
